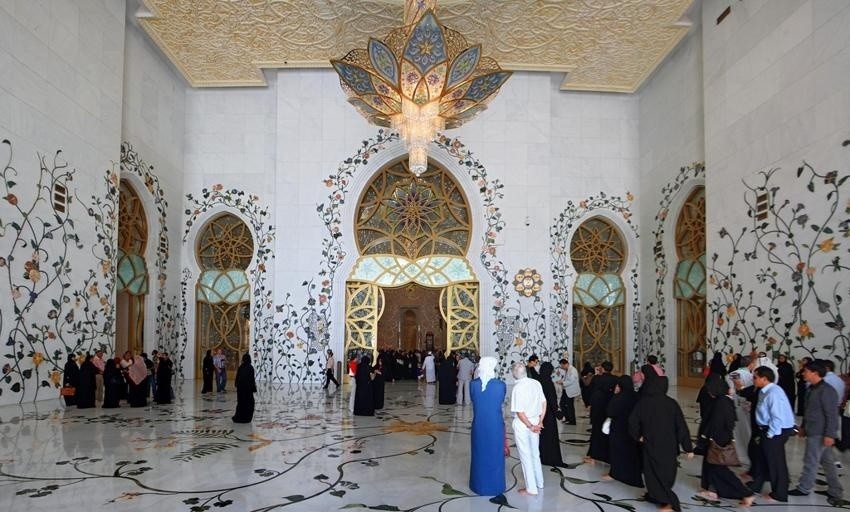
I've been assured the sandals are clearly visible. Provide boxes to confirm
[694,490,719,504]
[602,471,615,479]
[737,495,757,507]
[583,455,599,465]
[740,471,753,478]
[519,487,538,496]
[657,502,673,511]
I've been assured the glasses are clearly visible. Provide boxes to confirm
[753,375,763,380]
[534,360,539,364]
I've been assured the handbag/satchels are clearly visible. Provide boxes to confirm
[61,384,76,397]
[709,437,739,465]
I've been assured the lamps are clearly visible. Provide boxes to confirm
[330,0,514,177]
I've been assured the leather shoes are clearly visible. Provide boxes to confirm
[201,390,207,394]
[217,389,221,392]
[788,487,808,496]
[763,492,786,502]
[220,389,227,393]
[563,420,578,425]
[816,489,832,497]
[336,384,339,387]
[323,385,328,389]
[208,389,212,392]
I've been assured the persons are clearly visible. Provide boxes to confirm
[584,360,618,465]
[709,352,727,376]
[554,359,581,425]
[438,356,458,405]
[777,355,795,412]
[725,356,756,463]
[436,350,480,367]
[212,349,228,393]
[456,352,475,405]
[348,355,358,412]
[822,360,846,452]
[535,362,568,468]
[469,356,506,496]
[648,355,664,376]
[422,351,436,384]
[510,361,547,495]
[654,493,682,512]
[524,354,540,378]
[694,373,755,508]
[323,352,339,389]
[201,349,215,395]
[379,349,425,380]
[579,361,596,408]
[728,354,742,374]
[747,366,795,502]
[600,375,645,488]
[752,352,779,384]
[627,376,693,501]
[231,354,257,423]
[787,361,845,499]
[61,349,175,408]
[354,356,377,416]
[796,357,813,416]
[638,365,661,398]
[372,356,385,409]
